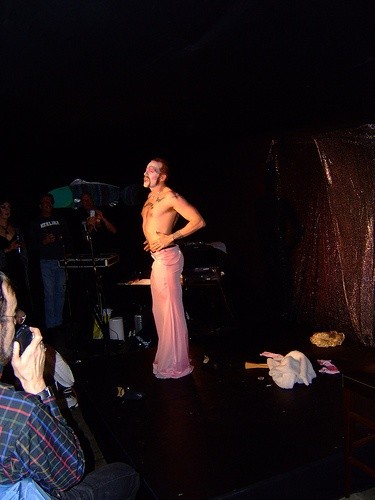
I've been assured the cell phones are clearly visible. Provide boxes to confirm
[90,210,95,218]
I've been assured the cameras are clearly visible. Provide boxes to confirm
[14,324,33,357]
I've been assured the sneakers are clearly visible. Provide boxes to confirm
[318,359,338,374]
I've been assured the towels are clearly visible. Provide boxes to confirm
[266,350,316,390]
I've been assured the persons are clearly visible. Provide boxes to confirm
[0,192,126,334]
[0,271,140,500]
[141,159,205,378]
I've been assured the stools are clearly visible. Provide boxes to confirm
[338,363,375,499]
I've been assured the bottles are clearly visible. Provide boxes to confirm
[16,234,23,254]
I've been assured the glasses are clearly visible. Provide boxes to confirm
[2,311,26,324]
